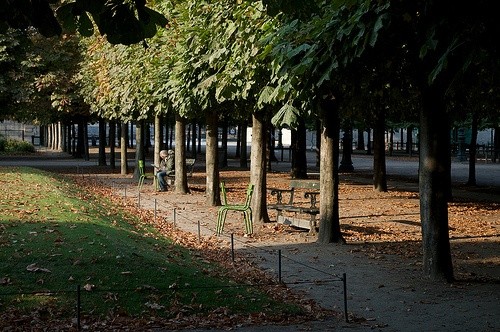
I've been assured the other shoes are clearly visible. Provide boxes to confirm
[156,187,168,192]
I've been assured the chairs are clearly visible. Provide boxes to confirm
[137,160,159,192]
[214,180,254,237]
[266,179,323,237]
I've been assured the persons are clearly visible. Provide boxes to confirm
[154,150,175,193]
